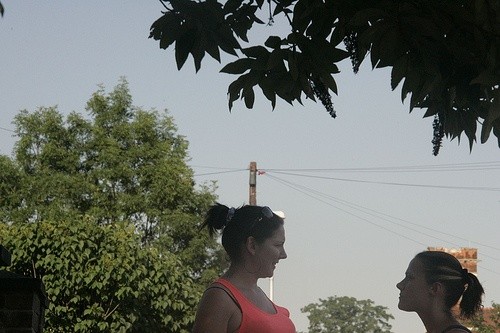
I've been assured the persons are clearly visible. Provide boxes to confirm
[396,251,484,333]
[192,202,298,333]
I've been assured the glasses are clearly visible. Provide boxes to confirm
[247,206,273,234]
